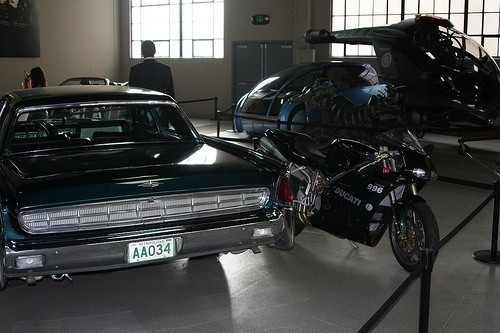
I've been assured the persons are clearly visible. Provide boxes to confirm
[23,66,49,122]
[129,40,175,129]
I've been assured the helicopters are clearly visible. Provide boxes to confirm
[231,13,500,133]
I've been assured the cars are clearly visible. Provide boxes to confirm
[0,76,291,289]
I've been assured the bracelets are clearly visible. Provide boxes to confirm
[25,79,29,82]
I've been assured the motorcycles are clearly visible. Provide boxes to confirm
[257,127,440,274]
